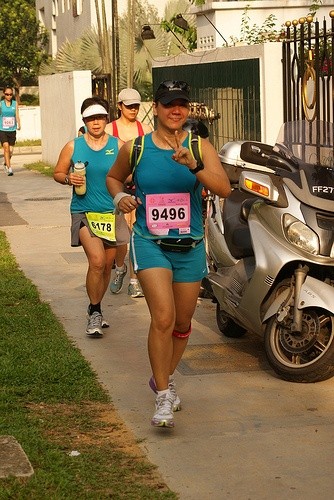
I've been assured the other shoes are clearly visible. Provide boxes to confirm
[4,164,13,176]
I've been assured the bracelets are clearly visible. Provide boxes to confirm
[113,192,131,209]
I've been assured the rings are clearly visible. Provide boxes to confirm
[129,204,132,207]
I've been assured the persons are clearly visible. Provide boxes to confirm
[0,87,21,176]
[106,80,232,427]
[77,98,110,137]
[106,87,152,298]
[53,97,125,337]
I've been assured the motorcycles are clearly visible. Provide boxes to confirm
[194,141,334,384]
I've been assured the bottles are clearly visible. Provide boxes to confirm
[73,159,87,196]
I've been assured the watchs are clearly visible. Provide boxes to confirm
[190,161,204,173]
[65,175,69,185]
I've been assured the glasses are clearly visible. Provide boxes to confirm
[4,93,13,96]
[157,80,191,95]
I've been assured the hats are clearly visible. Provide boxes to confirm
[118,88,141,106]
[82,104,109,118]
[155,82,191,105]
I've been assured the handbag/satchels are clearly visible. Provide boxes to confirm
[150,238,204,254]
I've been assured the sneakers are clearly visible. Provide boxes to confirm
[88,306,109,327]
[86,311,104,335]
[128,284,144,298]
[151,392,176,428]
[110,263,127,293]
[149,373,181,412]
